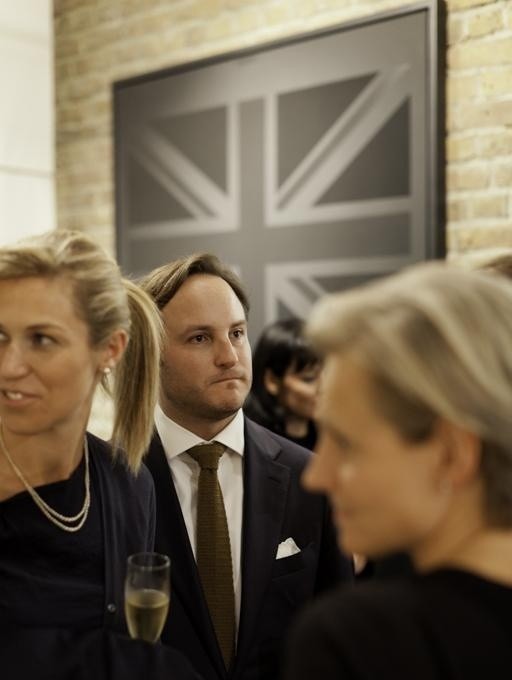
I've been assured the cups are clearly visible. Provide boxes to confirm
[124,550,172,646]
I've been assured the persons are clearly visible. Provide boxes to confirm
[1,227,197,679]
[271,260,511,679]
[133,251,357,680]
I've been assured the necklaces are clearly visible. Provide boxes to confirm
[1,432,91,532]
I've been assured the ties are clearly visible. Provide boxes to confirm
[184,441,238,670]
